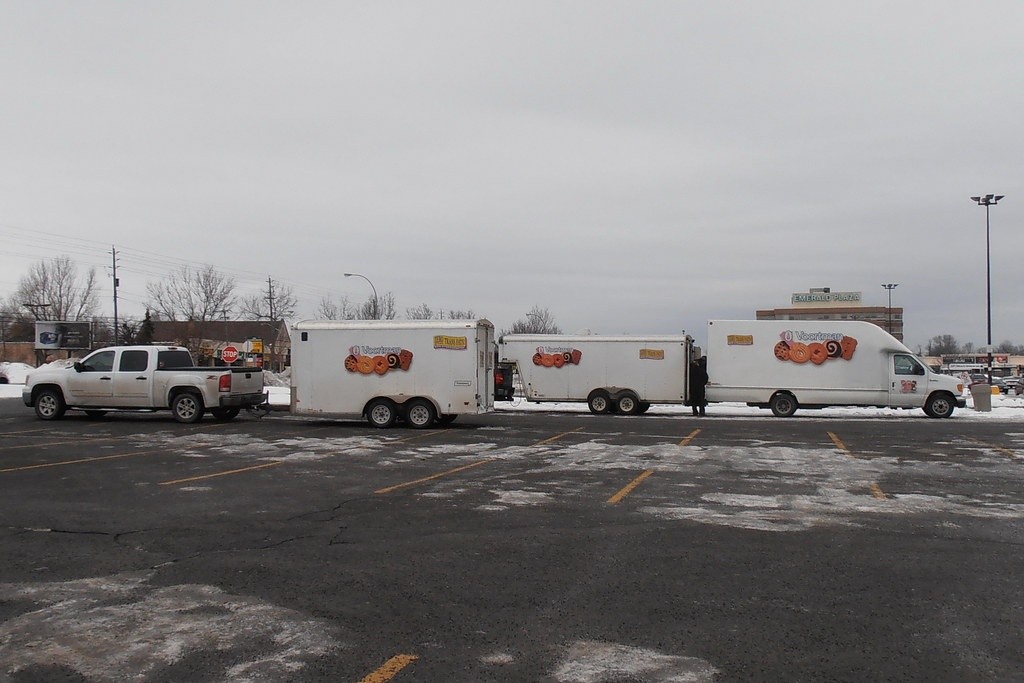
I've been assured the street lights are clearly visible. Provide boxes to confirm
[260,323,282,373]
[345,274,378,320]
[971,194,1004,385]
[526,314,546,334]
[882,283,898,333]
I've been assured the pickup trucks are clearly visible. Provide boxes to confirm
[21,347,266,424]
[494,369,515,401]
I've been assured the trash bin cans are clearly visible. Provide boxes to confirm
[494,364,515,401]
[972,384,992,412]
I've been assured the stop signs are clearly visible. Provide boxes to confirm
[222,347,239,363]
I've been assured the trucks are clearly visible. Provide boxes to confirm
[704,318,967,419]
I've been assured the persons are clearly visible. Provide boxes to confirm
[690,356,709,416]
[44,354,55,364]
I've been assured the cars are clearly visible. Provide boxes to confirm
[969,375,1024,394]
